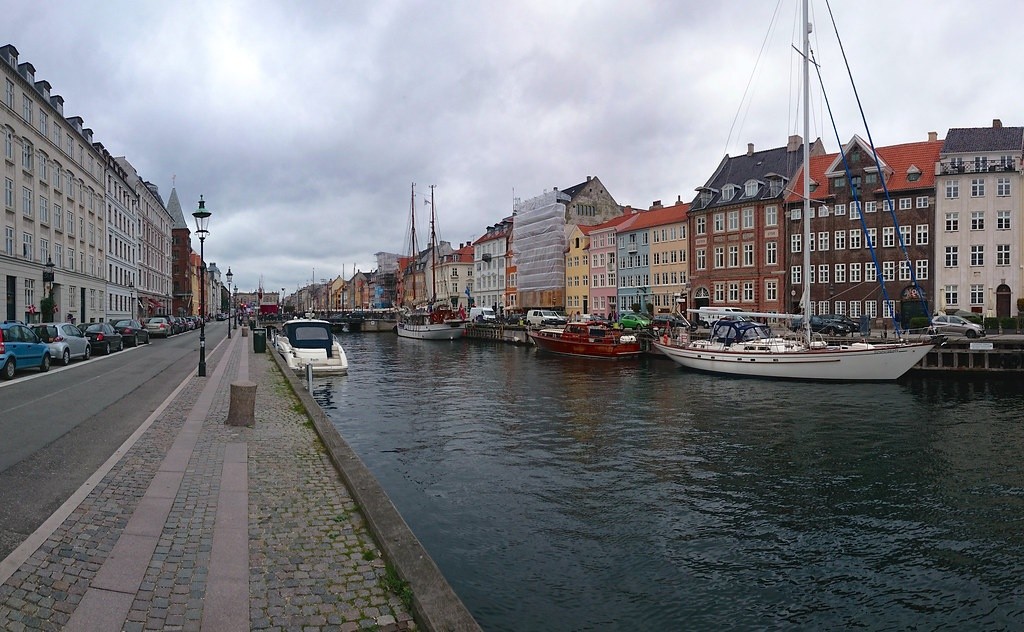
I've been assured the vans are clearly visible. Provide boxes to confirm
[469,307,497,322]
[699,306,755,329]
[526,309,573,327]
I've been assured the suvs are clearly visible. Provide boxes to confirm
[929,314,986,339]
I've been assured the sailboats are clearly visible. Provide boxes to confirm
[396,179,474,342]
[643,0,956,384]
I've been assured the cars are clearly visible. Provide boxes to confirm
[787,313,861,337]
[109,318,151,347]
[0,323,52,381]
[507,313,527,325]
[651,313,688,330]
[580,315,614,328]
[74,322,124,354]
[141,317,172,338]
[159,311,234,335]
[19,321,92,366]
[617,312,652,331]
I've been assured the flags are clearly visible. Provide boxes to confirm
[54,304,58,313]
[29,304,35,314]
[459,304,465,320]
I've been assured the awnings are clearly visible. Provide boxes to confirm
[142,296,166,308]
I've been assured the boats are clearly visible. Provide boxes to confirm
[526,308,645,360]
[270,304,350,379]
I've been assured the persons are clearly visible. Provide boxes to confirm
[614,312,619,322]
[259,311,267,321]
[902,312,912,334]
[576,312,581,322]
[608,313,612,321]
[893,310,901,333]
[239,313,243,326]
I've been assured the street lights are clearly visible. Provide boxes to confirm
[233,283,239,329]
[225,265,233,339]
[45,251,54,322]
[191,194,213,377]
